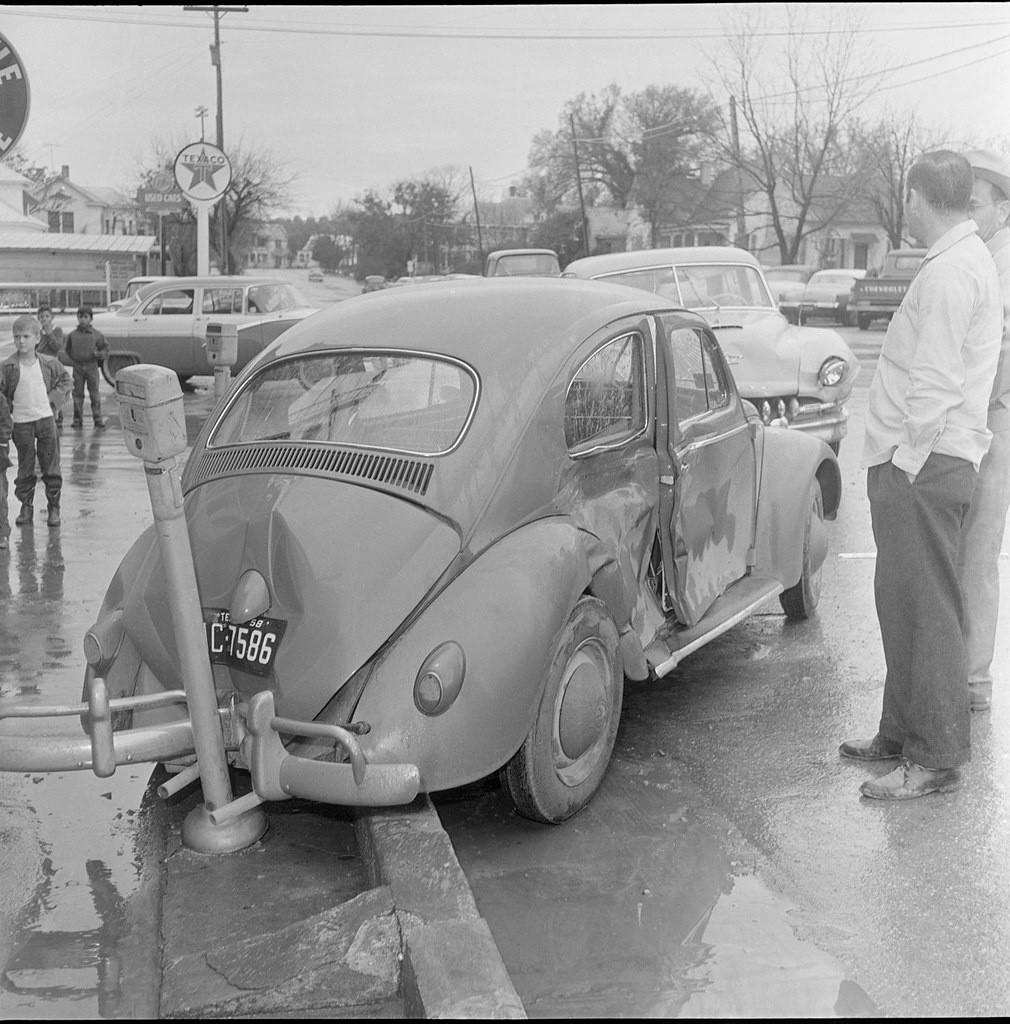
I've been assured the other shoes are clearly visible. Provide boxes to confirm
[969,680,992,710]
[0,536,9,548]
[56,420,62,427]
[47,507,61,526]
[16,504,33,523]
[95,421,105,427]
[71,421,82,427]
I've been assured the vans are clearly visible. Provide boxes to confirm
[482,249,562,281]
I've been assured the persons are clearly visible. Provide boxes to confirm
[0,306,111,549]
[839,151,1010,802]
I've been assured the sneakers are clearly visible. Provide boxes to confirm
[859,758,962,800]
[838,733,903,760]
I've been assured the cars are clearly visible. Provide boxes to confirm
[362,275,479,293]
[80,275,839,827]
[72,275,321,388]
[106,275,195,315]
[560,244,862,453]
[308,268,326,283]
[763,264,819,324]
[805,269,868,327]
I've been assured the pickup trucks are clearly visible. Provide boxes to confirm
[848,248,928,330]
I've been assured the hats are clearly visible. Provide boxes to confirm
[966,148,1010,201]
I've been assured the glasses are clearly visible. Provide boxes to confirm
[967,197,995,212]
